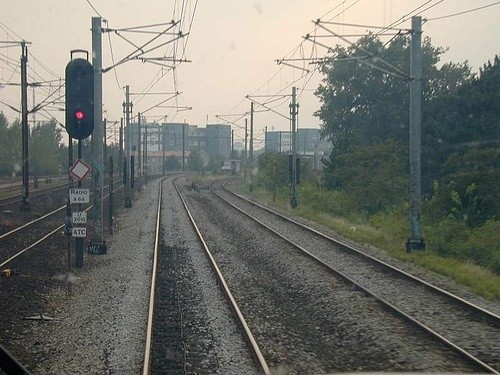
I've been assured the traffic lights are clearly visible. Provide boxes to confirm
[65,59,95,140]
